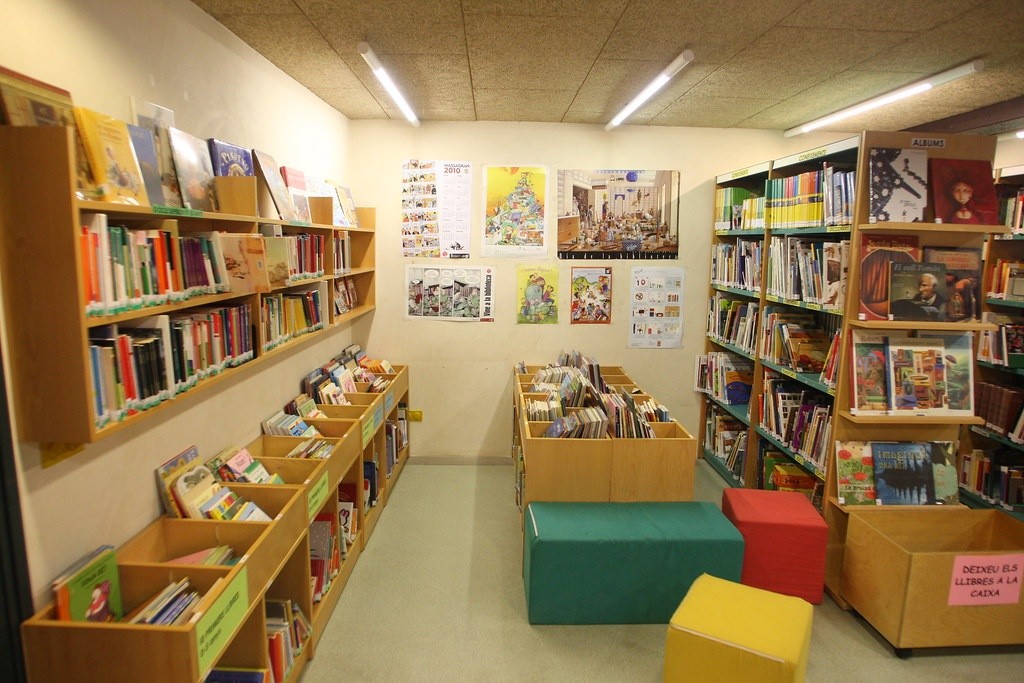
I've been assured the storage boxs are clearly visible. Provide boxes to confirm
[842,506,1024,658]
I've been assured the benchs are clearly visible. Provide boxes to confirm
[523,501,747,626]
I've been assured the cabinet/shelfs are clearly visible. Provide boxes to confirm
[692,129,1024,611]
[512,363,697,540]
[0,124,380,446]
[21,364,415,683]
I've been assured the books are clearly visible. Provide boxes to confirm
[0,66,408,683]
[517,147,1024,510]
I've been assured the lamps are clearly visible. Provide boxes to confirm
[604,48,695,132]
[356,40,420,127]
[782,58,986,138]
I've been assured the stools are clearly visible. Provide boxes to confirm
[659,573,814,683]
[720,486,830,607]
